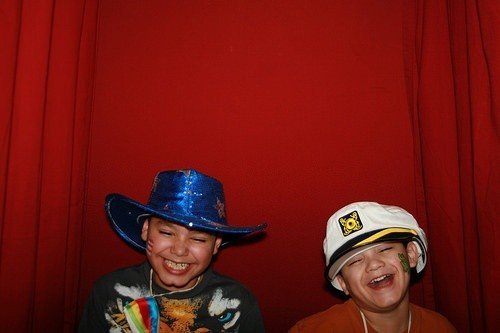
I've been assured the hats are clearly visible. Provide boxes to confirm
[322,201,428,291]
[104,169,269,256]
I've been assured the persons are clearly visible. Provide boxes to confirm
[284,200,459,333]
[77,167,267,333]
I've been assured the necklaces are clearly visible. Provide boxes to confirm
[144,267,202,298]
[354,303,412,333]
